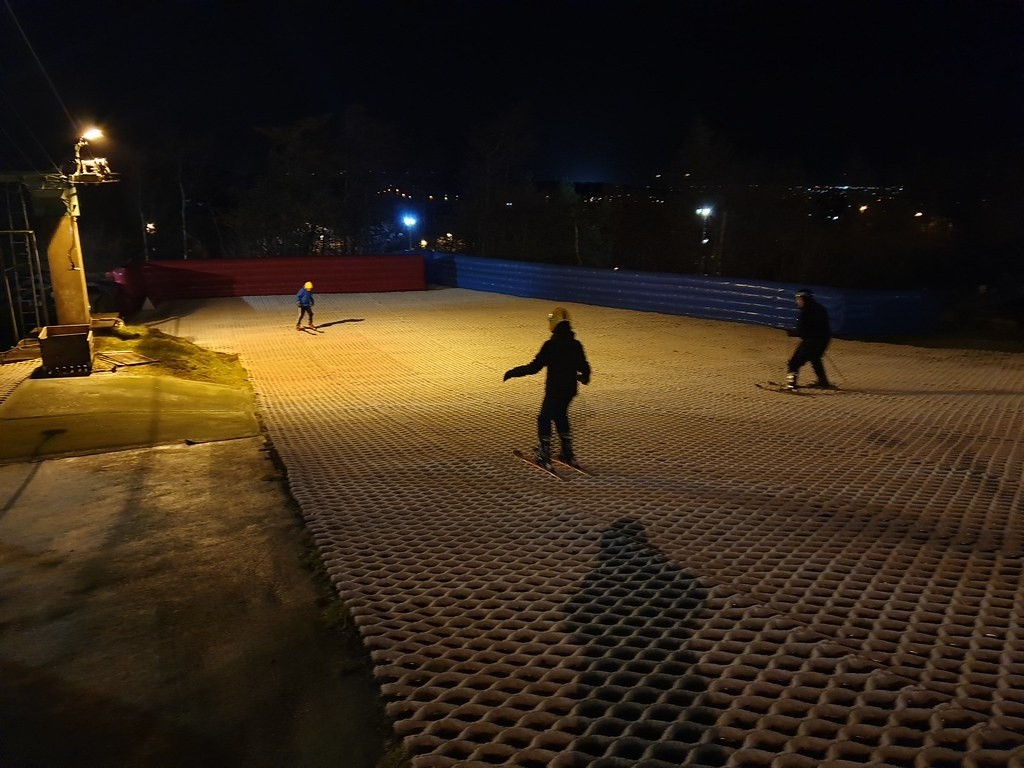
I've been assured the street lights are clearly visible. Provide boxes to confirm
[405,217,416,249]
[696,209,711,239]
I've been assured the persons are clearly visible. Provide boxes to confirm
[781,290,833,389]
[296,282,315,331]
[503,306,593,471]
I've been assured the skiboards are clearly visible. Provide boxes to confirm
[752,380,849,397]
[513,449,597,482]
[301,325,324,335]
